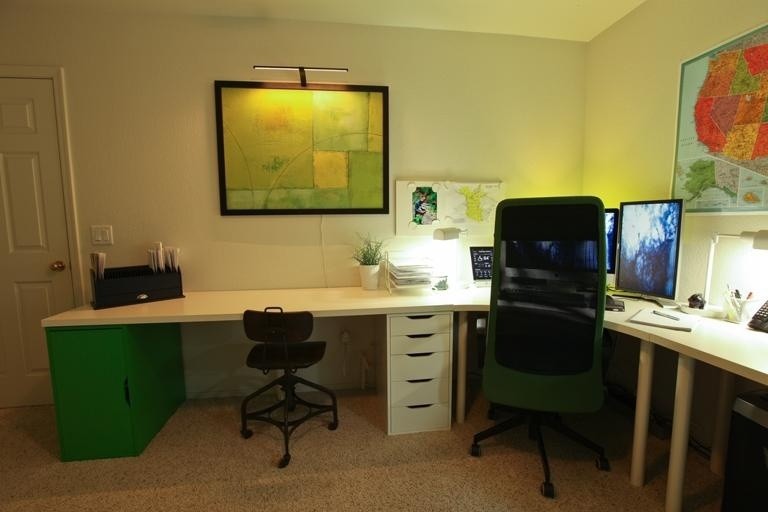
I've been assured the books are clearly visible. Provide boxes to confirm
[624,305,702,333]
[87,250,105,280]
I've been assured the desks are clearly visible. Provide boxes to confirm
[40,287,768,512]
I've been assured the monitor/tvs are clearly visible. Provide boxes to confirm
[612,200,682,308]
[605,205,619,276]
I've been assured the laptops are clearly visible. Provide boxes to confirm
[469,246,494,287]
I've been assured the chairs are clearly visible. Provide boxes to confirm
[472,195,612,498]
[240,306,337,467]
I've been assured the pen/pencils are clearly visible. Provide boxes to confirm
[654,310,680,321]
[724,284,753,322]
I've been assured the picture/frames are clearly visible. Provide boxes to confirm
[214,80,390,216]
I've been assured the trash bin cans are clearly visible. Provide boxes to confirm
[748,300,768,333]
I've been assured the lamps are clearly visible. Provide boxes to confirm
[254,65,349,87]
[431,226,468,241]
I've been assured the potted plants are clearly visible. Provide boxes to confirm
[349,232,385,289]
